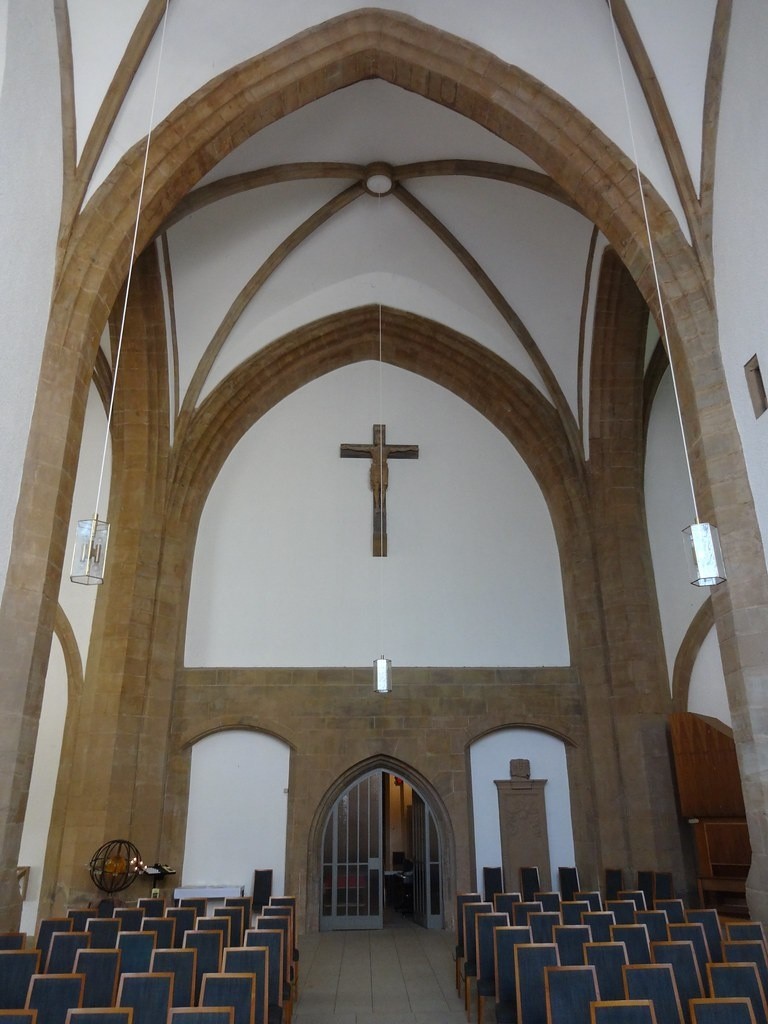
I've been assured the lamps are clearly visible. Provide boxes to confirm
[69,0,170,585]
[607,1,728,587]
[372,191,392,694]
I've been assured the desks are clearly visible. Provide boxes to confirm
[173,885,245,908]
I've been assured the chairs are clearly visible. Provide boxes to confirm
[0,869,300,1024]
[453,865,768,1024]
